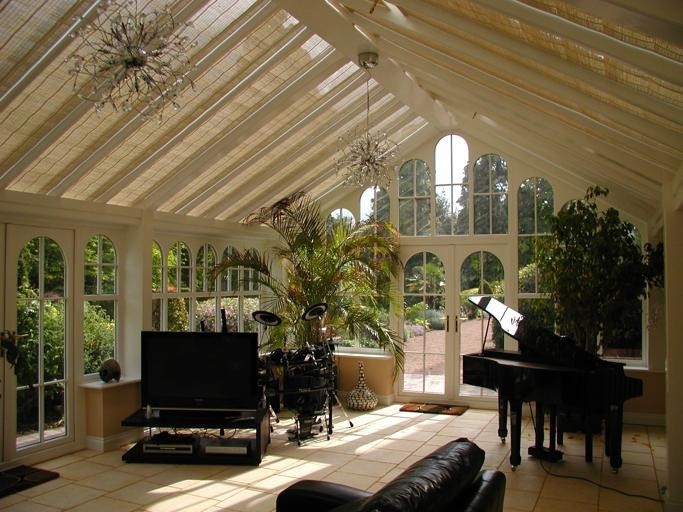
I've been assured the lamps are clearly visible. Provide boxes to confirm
[64,0,198,125]
[334,52,403,190]
[301,303,334,358]
[252,311,282,352]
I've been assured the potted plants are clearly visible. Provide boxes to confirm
[208,190,408,411]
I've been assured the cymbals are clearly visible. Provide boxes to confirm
[252,311,281,326]
[302,302,328,321]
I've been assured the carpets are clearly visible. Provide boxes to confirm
[0,465,59,499]
[400,402,469,415]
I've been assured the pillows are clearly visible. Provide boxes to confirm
[361,438,485,512]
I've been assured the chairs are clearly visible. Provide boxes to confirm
[276,437,506,512]
[268,342,353,446]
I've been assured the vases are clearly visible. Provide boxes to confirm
[346,361,378,411]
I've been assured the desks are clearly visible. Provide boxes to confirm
[121,409,271,466]
[80,377,144,453]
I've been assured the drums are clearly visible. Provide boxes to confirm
[322,342,335,352]
[290,349,307,362]
[282,366,327,413]
[318,345,330,358]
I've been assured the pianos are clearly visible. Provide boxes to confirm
[462,295,644,471]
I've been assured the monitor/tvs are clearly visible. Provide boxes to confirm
[141,330,258,412]
